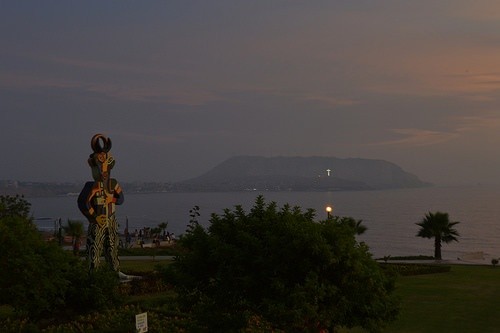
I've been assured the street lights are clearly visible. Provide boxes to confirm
[326,206,332,218]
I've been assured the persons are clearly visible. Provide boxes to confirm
[125,228,175,247]
